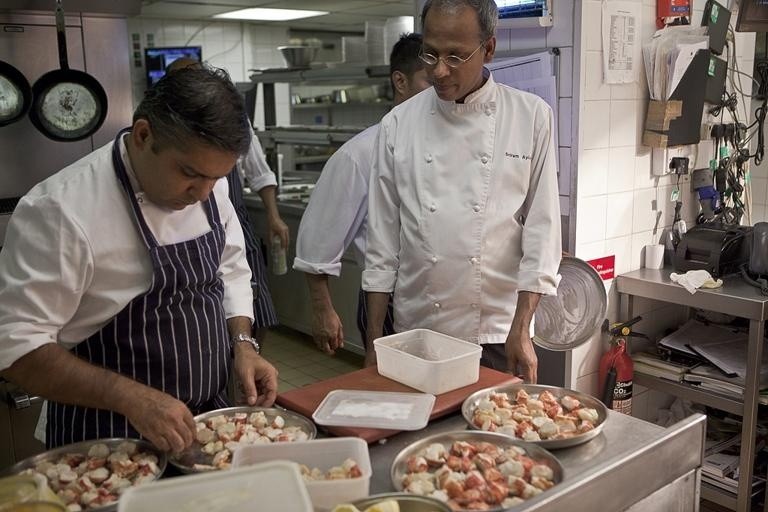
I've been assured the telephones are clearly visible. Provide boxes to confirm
[739,222,768,295]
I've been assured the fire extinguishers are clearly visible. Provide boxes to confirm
[597,314,649,415]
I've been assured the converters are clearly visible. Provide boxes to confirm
[672,158,689,174]
[723,124,731,137]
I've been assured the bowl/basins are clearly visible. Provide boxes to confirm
[277,42,322,68]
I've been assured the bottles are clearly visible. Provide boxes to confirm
[271,232,287,275]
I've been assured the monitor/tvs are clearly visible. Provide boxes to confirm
[144,46,202,89]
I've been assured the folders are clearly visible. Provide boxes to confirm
[642,47,710,150]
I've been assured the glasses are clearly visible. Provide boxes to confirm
[419,40,487,66]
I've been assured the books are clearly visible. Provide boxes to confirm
[630,316,768,405]
[700,413,768,496]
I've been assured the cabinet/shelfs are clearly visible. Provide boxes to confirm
[317,383,707,512]
[242,64,397,358]
[617,261,768,511]
[0,8,133,252]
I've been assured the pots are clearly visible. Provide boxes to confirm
[1,60,34,126]
[28,0,110,143]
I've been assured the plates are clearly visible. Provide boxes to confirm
[461,382,611,447]
[389,429,566,512]
[164,403,316,477]
[5,436,167,511]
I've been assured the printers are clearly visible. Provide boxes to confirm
[674,223,754,279]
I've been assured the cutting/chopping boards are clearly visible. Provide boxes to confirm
[276,363,524,446]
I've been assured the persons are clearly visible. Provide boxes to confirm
[362,1,562,383]
[294,36,427,354]
[1,66,277,454]
[165,57,293,358]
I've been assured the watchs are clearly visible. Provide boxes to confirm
[232,331,263,352]
